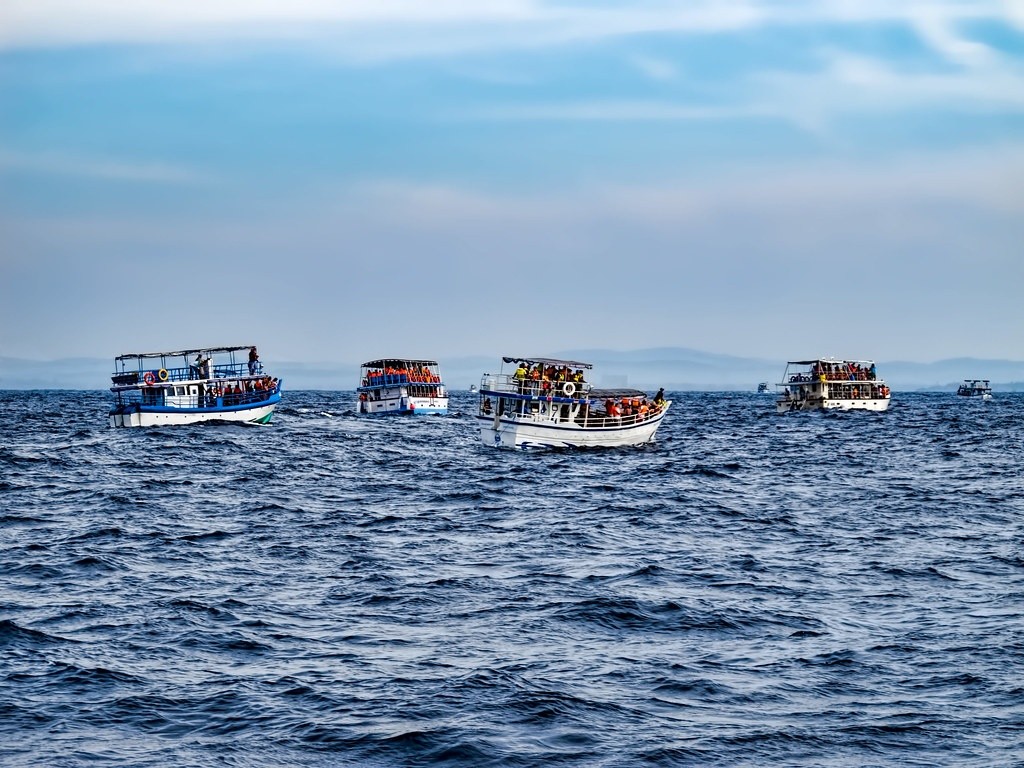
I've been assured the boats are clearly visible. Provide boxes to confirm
[356,359,449,415]
[774,359,891,412]
[757,382,770,393]
[957,379,992,399]
[110,346,283,425]
[480,356,672,446]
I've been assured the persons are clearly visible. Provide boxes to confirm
[602,387,667,426]
[224,384,232,406]
[385,365,407,375]
[788,361,877,382]
[408,386,438,398]
[782,388,791,400]
[206,385,222,408]
[359,392,373,402]
[189,354,211,380]
[851,384,890,399]
[407,365,440,383]
[248,347,259,376]
[512,363,588,399]
[245,375,278,404]
[234,385,242,405]
[482,398,492,415]
[362,369,382,387]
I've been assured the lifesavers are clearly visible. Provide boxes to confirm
[562,382,576,396]
[144,373,155,385]
[411,377,440,383]
[158,369,168,380]
